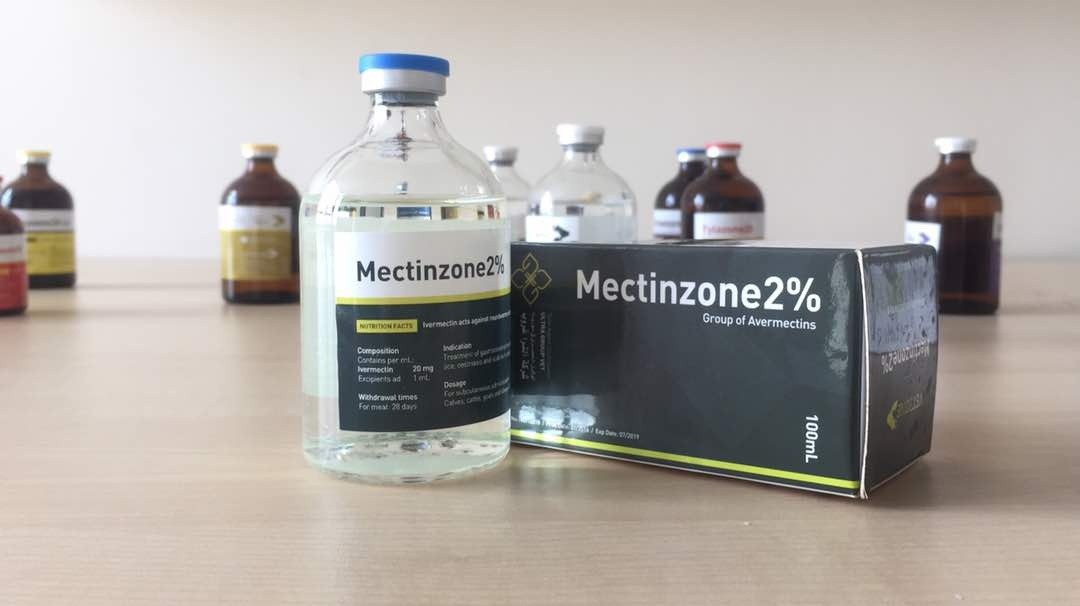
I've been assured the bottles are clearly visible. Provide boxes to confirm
[904,136,1004,316]
[650,147,707,242]
[525,123,639,244]
[679,143,765,243]
[0,174,28,315]
[476,140,531,244]
[219,143,301,305]
[299,53,514,488]
[0,151,75,288]
